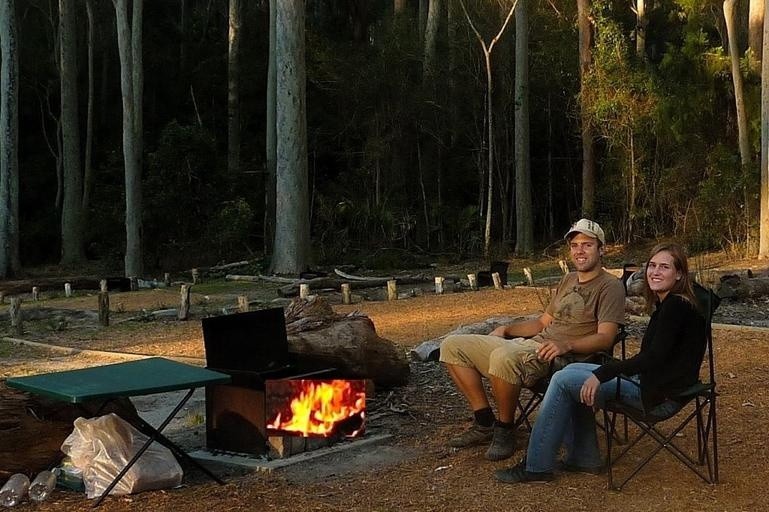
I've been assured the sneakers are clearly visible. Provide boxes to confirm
[561,457,601,475]
[451,421,495,447]
[494,455,554,483]
[485,427,517,460]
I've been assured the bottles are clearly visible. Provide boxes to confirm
[0,467,60,507]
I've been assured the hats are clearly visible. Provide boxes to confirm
[564,219,606,245]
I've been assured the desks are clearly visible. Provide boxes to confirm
[4,354,234,506]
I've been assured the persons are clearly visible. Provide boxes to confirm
[492,242,709,483]
[438,217,625,461]
